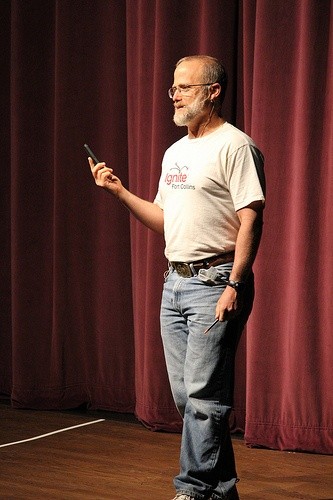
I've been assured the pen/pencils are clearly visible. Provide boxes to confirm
[203,318,219,334]
[83,144,99,165]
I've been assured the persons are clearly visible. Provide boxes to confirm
[86,53,266,500]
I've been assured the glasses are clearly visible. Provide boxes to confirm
[168,83,210,98]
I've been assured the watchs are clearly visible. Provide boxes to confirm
[226,279,241,291]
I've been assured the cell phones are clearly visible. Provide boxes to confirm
[83,144,103,170]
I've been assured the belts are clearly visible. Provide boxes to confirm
[170,252,234,278]
